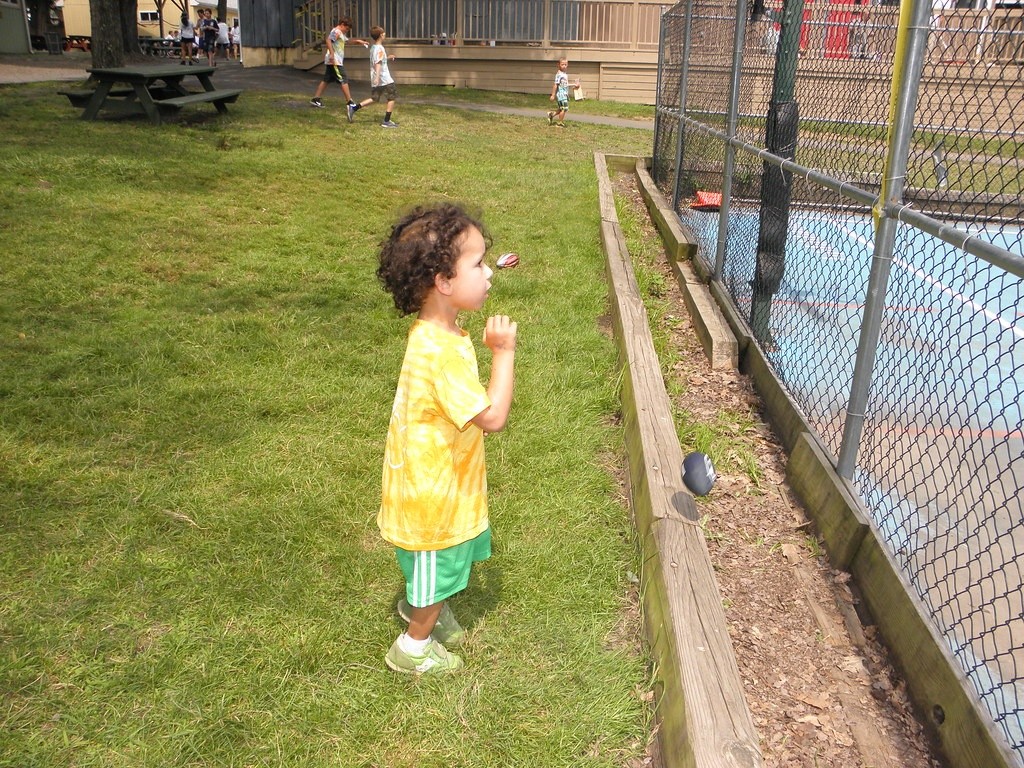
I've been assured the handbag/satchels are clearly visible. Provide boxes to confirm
[573,85,584,101]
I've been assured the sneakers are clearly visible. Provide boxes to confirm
[397,596,465,646]
[346,105,354,123]
[381,120,398,128]
[310,97,326,108]
[346,99,358,108]
[385,634,464,676]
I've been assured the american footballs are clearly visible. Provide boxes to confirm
[496,252,520,269]
[680,451,718,497]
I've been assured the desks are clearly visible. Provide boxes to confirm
[66,36,88,52]
[141,38,175,46]
[82,64,228,120]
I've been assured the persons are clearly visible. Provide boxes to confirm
[162,7,240,69]
[846,8,872,59]
[310,15,369,108]
[347,26,398,128]
[547,58,580,128]
[377,201,516,678]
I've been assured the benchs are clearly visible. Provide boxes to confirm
[56,86,162,107]
[154,89,242,115]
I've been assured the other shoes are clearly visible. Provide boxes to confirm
[556,122,565,128]
[547,112,553,125]
[181,61,185,65]
[189,62,193,65]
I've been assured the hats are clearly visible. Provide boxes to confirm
[180,12,188,18]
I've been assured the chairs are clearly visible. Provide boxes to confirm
[65,34,181,57]
